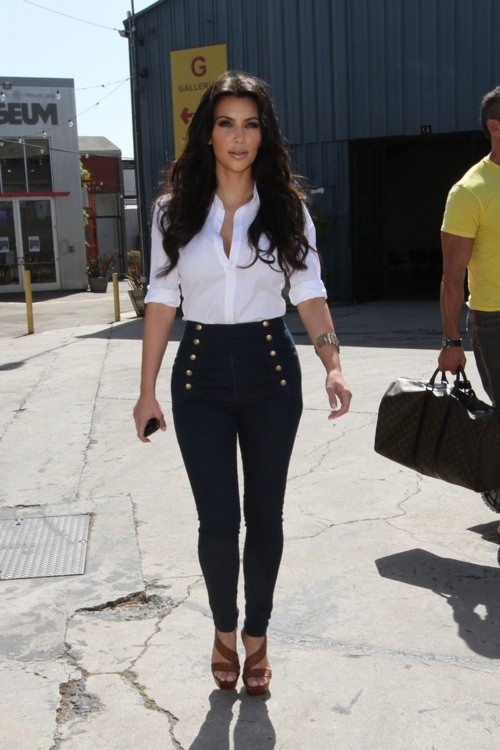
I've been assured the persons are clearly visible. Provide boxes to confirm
[438,86,500,560]
[133,71,352,695]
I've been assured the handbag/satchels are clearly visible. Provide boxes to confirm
[373,362,500,494]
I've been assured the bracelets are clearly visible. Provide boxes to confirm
[314,332,340,355]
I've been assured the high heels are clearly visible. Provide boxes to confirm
[211,627,240,690]
[241,626,272,697]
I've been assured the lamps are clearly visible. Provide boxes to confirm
[3,81,12,90]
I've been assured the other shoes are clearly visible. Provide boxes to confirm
[480,486,500,514]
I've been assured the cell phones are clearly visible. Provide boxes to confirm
[145,418,159,437]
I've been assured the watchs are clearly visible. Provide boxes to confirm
[440,338,461,348]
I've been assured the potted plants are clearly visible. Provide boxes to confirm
[122,250,145,317]
[84,249,120,293]
